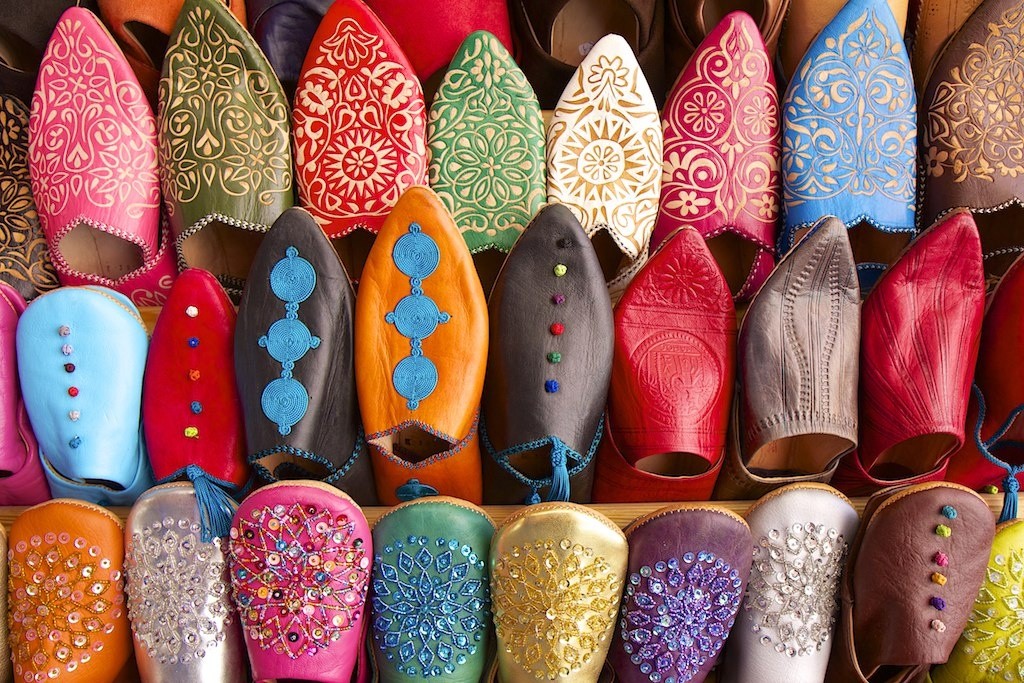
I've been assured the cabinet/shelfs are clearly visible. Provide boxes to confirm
[0,109,1024,543]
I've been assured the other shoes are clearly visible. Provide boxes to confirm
[1,0,1024,683]
